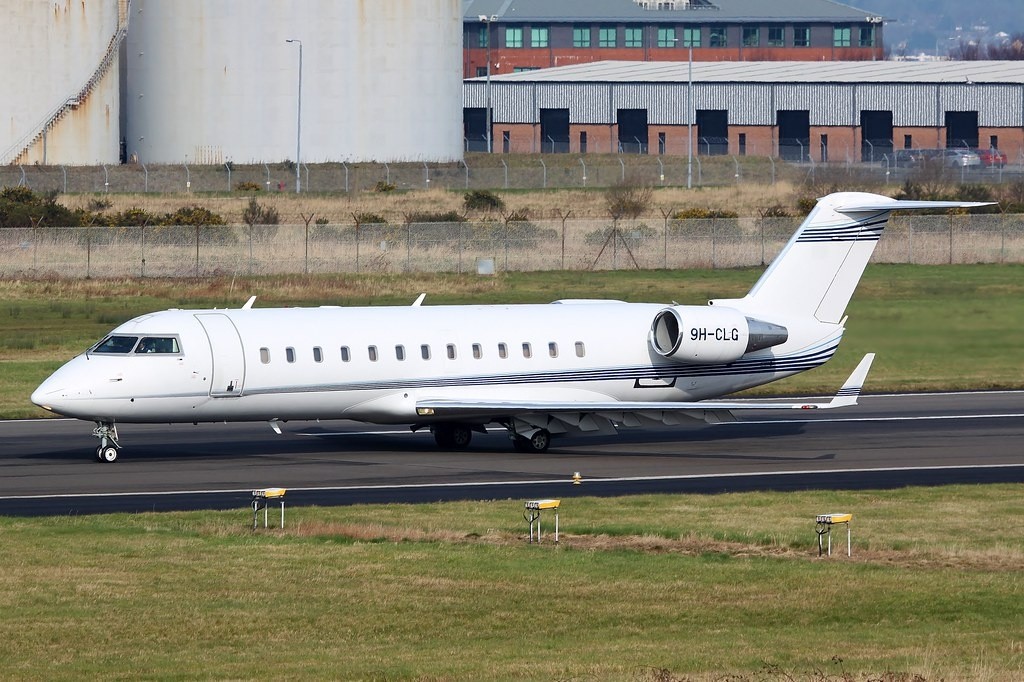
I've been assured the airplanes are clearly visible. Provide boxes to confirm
[29,189,999,462]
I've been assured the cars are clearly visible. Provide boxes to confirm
[881,149,940,169]
[929,147,981,169]
[970,148,1008,168]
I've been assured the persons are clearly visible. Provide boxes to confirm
[134,342,151,353]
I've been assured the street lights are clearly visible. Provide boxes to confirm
[669,39,696,189]
[285,38,304,195]
[478,13,499,154]
